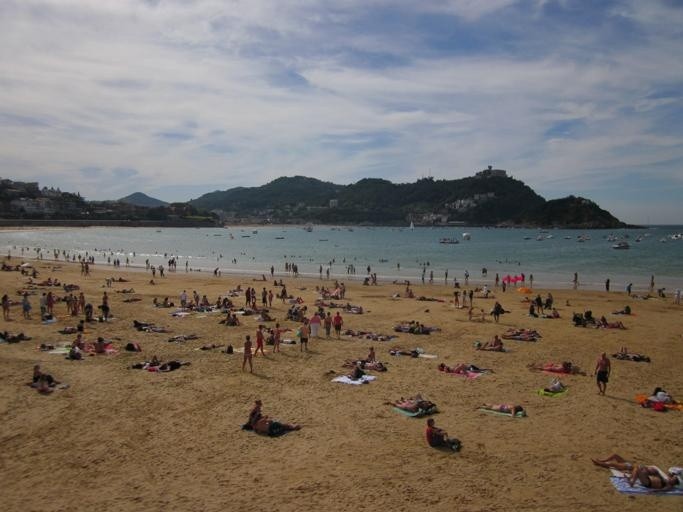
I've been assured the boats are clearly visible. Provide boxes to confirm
[406,225,682,251]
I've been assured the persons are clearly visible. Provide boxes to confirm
[476,402,526,417]
[594,352,610,396]
[625,466,679,495]
[425,418,448,448]
[37,377,49,392]
[406,391,433,410]
[591,454,658,475]
[653,387,673,404]
[383,400,418,415]
[544,377,566,394]
[32,365,62,384]
[333,361,360,379]
[248,400,263,421]
[1,244,683,374]
[243,415,302,436]
